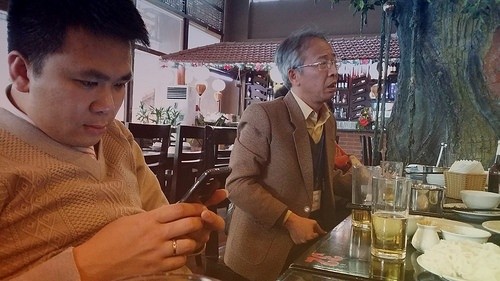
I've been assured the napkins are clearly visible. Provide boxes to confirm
[448,159,485,174]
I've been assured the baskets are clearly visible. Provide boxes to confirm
[442,169,486,199]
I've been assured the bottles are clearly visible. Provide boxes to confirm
[411,223,440,253]
[488,140,500,193]
[405,215,422,236]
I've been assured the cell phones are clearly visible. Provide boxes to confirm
[177,167,231,204]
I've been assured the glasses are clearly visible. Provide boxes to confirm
[293,59,341,71]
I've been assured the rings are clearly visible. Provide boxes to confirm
[172,239,177,256]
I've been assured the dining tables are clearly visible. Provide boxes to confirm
[277,196,500,281]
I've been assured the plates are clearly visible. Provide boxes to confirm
[425,216,472,235]
[416,254,466,281]
[453,210,500,219]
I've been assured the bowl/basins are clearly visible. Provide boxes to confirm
[439,226,491,244]
[460,189,500,209]
[410,183,446,215]
[427,173,446,187]
[482,220,500,243]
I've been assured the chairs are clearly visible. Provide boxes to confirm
[126,123,169,196]
[170,123,205,203]
[205,123,238,260]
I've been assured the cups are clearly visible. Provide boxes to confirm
[380,160,404,179]
[351,164,381,232]
[370,175,412,265]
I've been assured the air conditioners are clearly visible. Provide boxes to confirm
[167,86,196,145]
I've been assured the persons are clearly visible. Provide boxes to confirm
[273,85,350,172]
[224,31,368,281]
[0,0,228,281]
